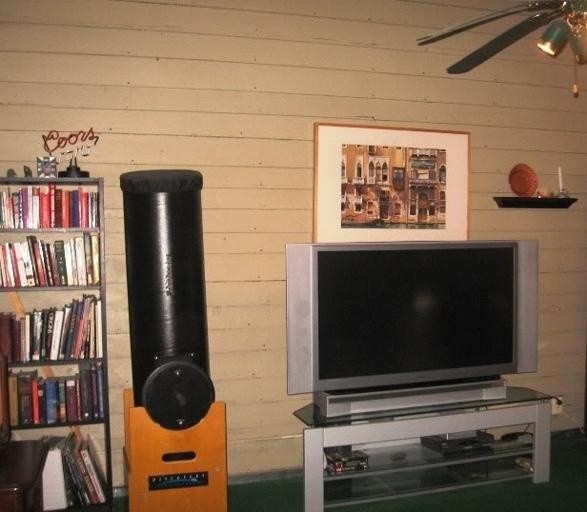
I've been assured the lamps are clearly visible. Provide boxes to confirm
[535,10,587,96]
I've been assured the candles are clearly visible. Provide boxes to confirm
[558,166,565,192]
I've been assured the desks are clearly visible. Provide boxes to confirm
[0,438,52,512]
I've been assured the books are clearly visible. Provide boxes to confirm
[0,182,105,512]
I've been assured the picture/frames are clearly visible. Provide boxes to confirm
[309,118,476,248]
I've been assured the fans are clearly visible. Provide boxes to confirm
[414,1,587,76]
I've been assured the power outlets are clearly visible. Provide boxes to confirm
[547,390,564,417]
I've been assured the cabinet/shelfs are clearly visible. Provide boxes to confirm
[0,170,115,508]
[285,383,559,512]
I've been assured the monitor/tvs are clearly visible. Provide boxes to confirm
[284,238,541,420]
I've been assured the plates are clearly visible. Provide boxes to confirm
[507,163,537,197]
